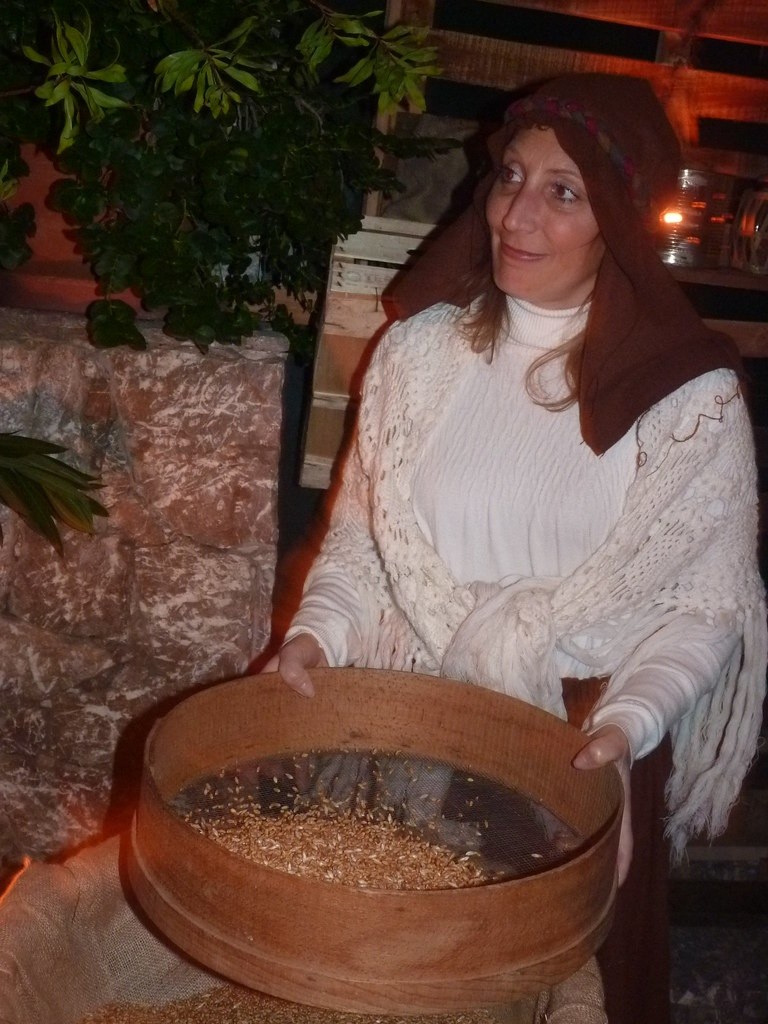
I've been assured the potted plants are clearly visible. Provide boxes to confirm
[0,0,467,365]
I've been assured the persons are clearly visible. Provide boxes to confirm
[256,73,768,1024]
